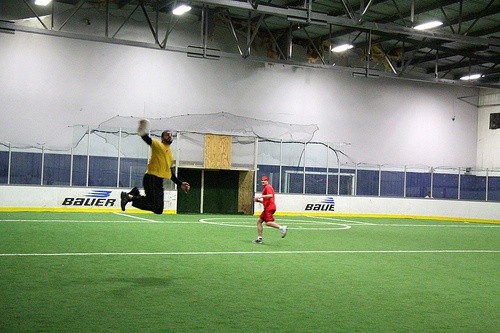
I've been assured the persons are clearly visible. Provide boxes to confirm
[120,118,191,215]
[252,175,288,246]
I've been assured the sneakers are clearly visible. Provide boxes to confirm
[130,187,139,197]
[281,225,289,238]
[121,191,130,211]
[253,237,263,244]
